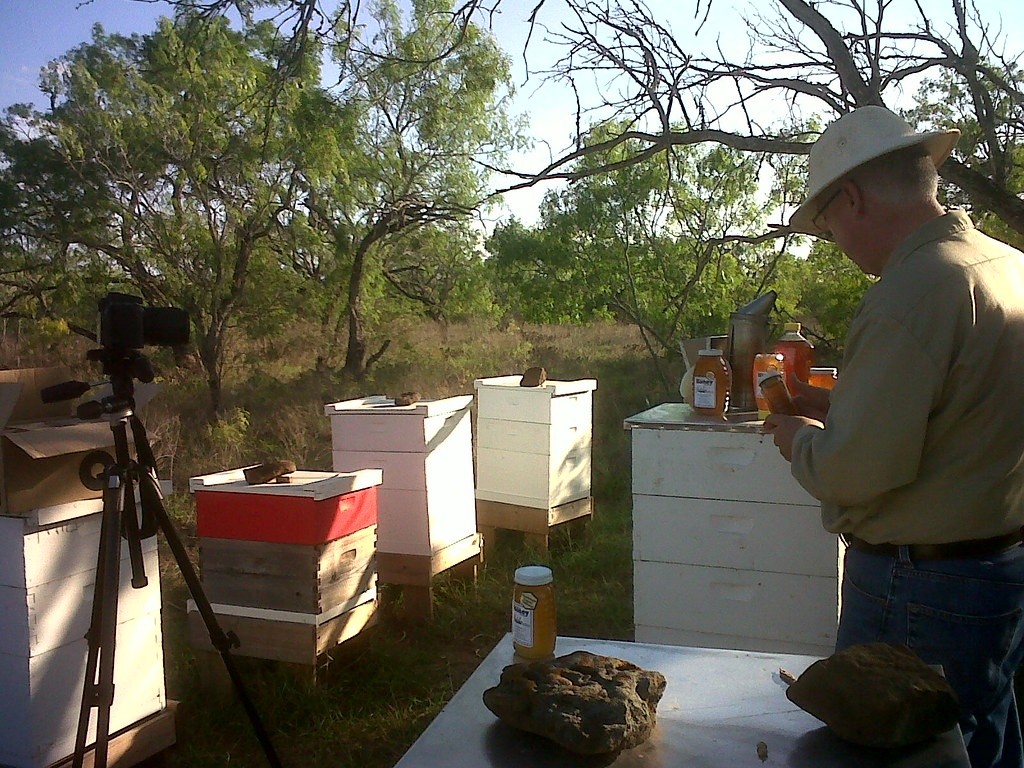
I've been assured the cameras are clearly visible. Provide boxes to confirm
[98,292,190,350]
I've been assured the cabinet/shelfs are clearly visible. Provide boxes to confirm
[623,401,844,657]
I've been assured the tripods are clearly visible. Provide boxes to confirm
[39,347,285,768]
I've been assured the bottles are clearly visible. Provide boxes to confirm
[808,367,838,392]
[513,566,555,659]
[758,371,801,416]
[752,353,786,420]
[773,322,816,397]
[692,350,729,417]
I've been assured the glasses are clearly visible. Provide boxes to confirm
[812,178,856,241]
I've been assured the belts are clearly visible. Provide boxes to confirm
[838,529,1021,560]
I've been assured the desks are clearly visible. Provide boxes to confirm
[393,629,971,768]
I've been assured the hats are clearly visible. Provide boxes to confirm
[789,105,961,236]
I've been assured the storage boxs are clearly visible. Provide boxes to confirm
[185,374,597,696]
[0,481,180,768]
[0,364,138,516]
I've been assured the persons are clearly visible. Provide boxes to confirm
[759,106,1024,768]
